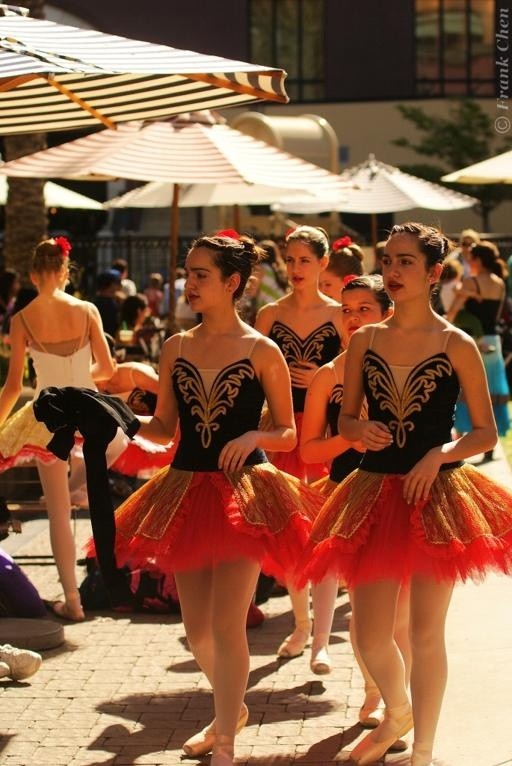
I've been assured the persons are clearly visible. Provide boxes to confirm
[296,223,510,766]
[299,278,413,720]
[89,235,330,766]
[1,644,43,681]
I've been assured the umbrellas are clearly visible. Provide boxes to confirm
[2,111,373,335]
[441,148,511,185]
[102,181,371,233]
[267,153,481,248]
[1,3,288,135]
[3,177,102,214]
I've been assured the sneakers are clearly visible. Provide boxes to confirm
[347,699,434,766]
[182,702,249,766]
[277,613,313,659]
[0,644,43,682]
[357,679,385,727]
[310,634,331,675]
[53,591,86,622]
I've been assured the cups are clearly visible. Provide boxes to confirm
[119,330,134,346]
